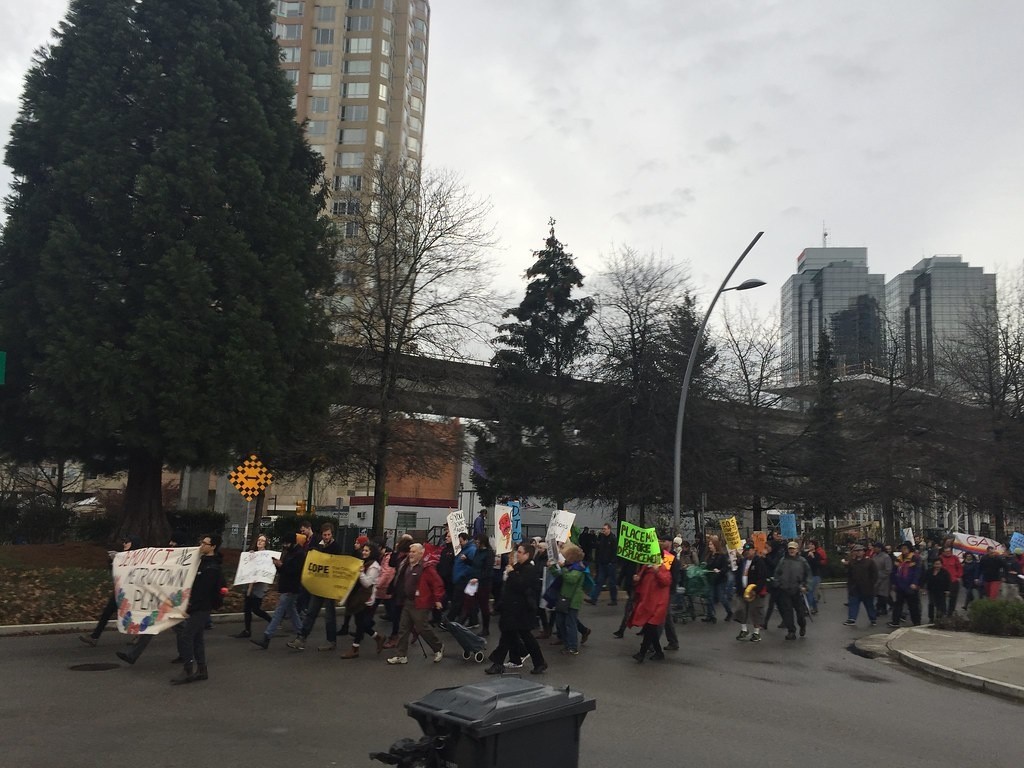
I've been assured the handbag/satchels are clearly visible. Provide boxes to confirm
[556,595,571,613]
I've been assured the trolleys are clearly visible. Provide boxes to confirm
[672,561,718,624]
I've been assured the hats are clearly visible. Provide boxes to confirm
[661,531,1023,562]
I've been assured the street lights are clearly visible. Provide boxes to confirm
[673,232,767,527]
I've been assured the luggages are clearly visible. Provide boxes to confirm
[436,606,487,663]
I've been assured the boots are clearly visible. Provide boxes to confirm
[410,624,421,644]
[371,632,386,654]
[341,643,359,659]
[336,624,350,635]
[383,635,399,648]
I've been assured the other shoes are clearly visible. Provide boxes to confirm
[538,586,978,662]
[250,637,270,649]
[209,623,215,629]
[465,621,480,628]
[287,638,307,650]
[504,662,523,668]
[115,651,134,664]
[318,640,337,651]
[520,653,531,663]
[530,661,548,674]
[79,635,98,648]
[485,663,506,674]
[185,668,209,681]
[171,656,185,664]
[379,615,392,622]
[235,630,251,638]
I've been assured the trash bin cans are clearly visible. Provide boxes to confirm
[404,671,596,768]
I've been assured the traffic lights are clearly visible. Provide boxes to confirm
[296,500,307,516]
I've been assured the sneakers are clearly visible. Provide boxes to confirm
[387,656,408,663]
[433,643,445,663]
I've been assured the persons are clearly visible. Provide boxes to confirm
[239,509,618,668]
[844,550,877,626]
[843,536,1024,624]
[887,544,922,627]
[170,535,222,684]
[626,550,672,661]
[484,543,547,675]
[613,532,827,650]
[116,536,187,664]
[78,535,139,648]
